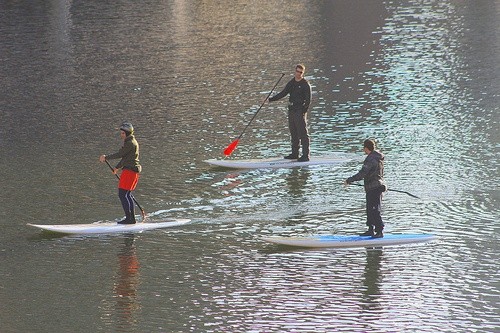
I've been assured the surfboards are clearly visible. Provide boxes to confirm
[202,154,345,169]
[26,218,192,235]
[257,233,434,248]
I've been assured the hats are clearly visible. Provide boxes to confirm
[363,138,375,150]
[119,122,134,133]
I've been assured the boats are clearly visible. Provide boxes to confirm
[25,218,191,234]
[258,233,442,248]
[202,156,353,168]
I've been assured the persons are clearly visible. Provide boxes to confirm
[100,122,142,225]
[266,63,313,162]
[344,138,386,239]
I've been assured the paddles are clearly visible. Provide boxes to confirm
[223,73,286,156]
[346,182,423,201]
[105,158,146,221]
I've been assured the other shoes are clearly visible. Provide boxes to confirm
[284,154,299,159]
[359,230,374,236]
[372,232,384,238]
[297,156,310,162]
[117,218,131,224]
[131,218,136,224]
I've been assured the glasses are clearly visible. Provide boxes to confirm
[296,69,304,74]
